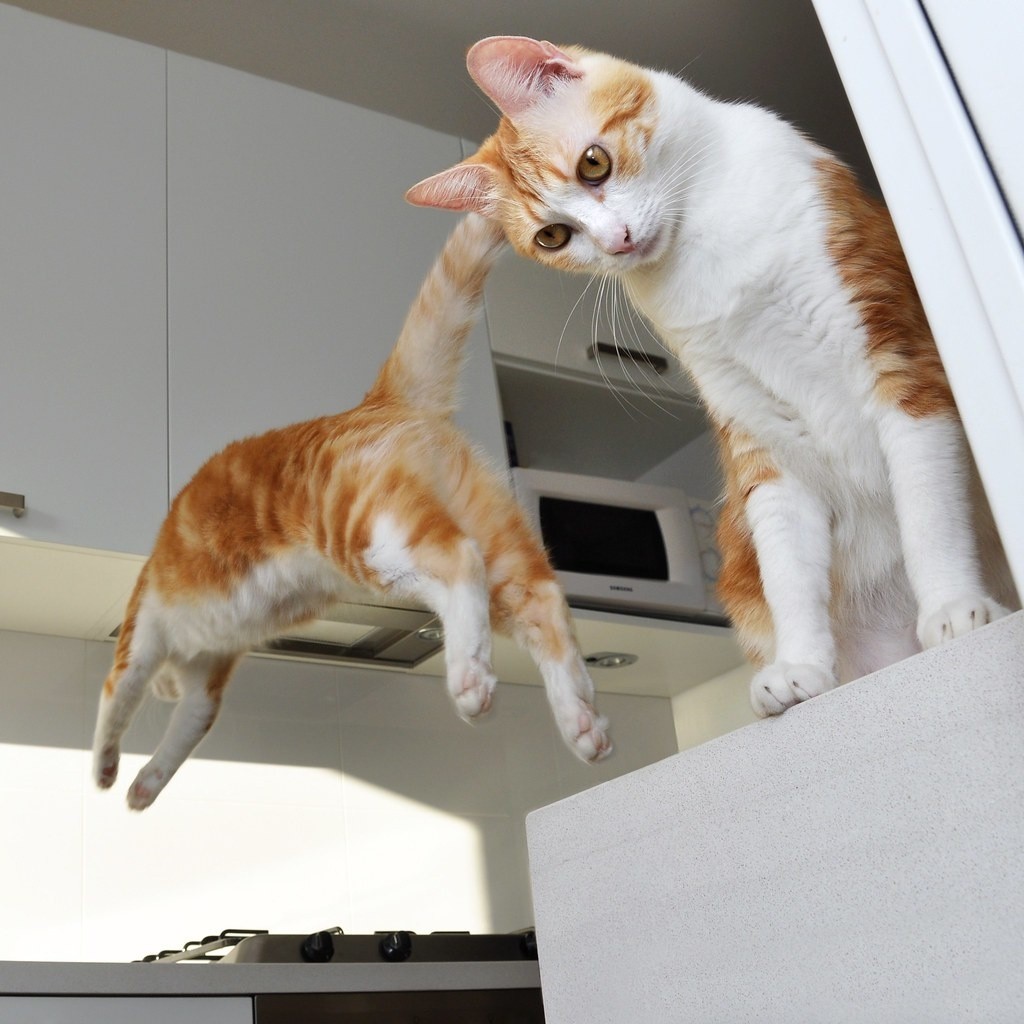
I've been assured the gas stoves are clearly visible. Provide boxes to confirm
[129,927,537,962]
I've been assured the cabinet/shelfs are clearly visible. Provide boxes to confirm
[461,139,719,482]
[0,986,546,1024]
[0,0,517,671]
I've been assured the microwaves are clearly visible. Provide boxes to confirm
[510,469,729,625]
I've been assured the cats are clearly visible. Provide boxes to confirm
[91,32,1022,811]
